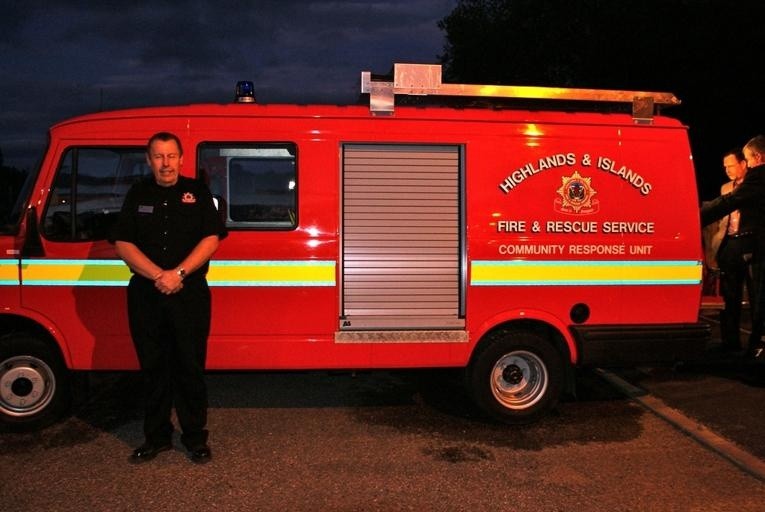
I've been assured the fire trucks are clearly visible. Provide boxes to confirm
[0,61,729,434]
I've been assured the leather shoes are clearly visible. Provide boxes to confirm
[182,438,211,463]
[133,439,172,461]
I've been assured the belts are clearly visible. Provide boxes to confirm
[726,234,739,238]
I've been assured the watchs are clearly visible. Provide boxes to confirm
[175,266,186,280]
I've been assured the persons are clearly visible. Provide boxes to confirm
[710,150,750,352]
[107,131,230,462]
[699,134,765,347]
